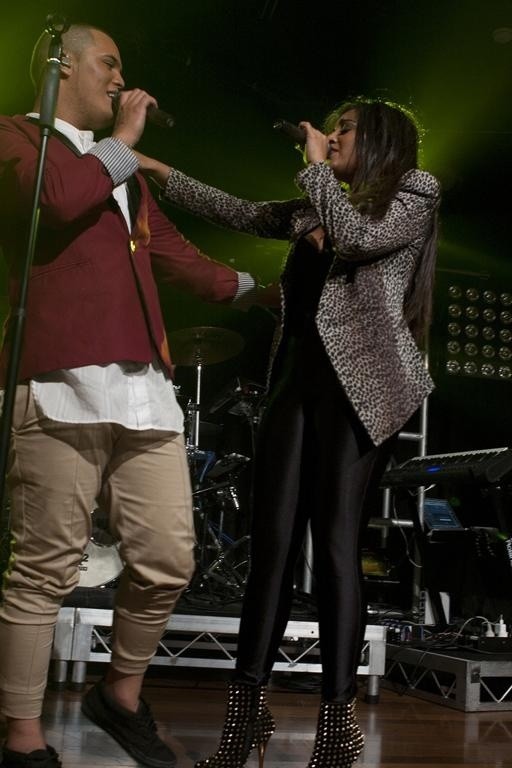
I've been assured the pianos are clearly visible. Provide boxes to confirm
[378,448,511,484]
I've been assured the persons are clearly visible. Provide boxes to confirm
[124,93,442,767]
[3,19,284,768]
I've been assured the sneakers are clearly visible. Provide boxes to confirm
[0,744,65,767]
[81,685,179,765]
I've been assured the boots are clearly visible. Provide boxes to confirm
[196,687,276,768]
[308,698,366,768]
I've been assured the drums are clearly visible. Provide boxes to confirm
[64,503,127,606]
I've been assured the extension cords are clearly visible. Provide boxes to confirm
[473,636,512,652]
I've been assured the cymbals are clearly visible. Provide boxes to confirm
[165,325,244,367]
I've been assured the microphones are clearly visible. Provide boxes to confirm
[112,93,176,130]
[273,119,306,145]
